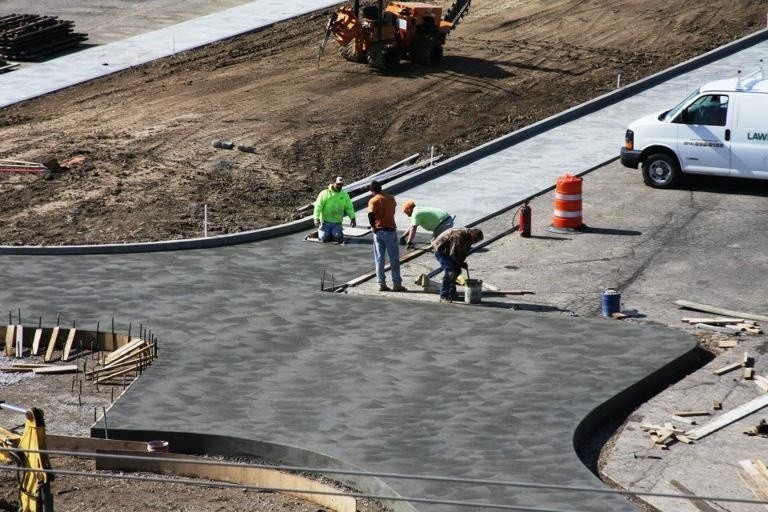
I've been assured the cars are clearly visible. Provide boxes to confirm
[620,59,767,187]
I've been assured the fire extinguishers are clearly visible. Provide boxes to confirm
[512,199,531,237]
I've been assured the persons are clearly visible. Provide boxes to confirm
[366,181,408,293]
[399,199,456,249]
[313,174,357,244]
[429,226,484,305]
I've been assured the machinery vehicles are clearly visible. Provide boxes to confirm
[315,0,472,75]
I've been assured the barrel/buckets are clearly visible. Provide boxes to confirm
[602,293,621,317]
[464,279,483,304]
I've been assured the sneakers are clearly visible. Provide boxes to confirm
[441,298,452,305]
[379,285,406,292]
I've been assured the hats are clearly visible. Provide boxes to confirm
[402,202,413,211]
[336,177,343,184]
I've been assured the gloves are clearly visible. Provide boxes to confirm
[407,241,412,250]
[400,236,406,245]
